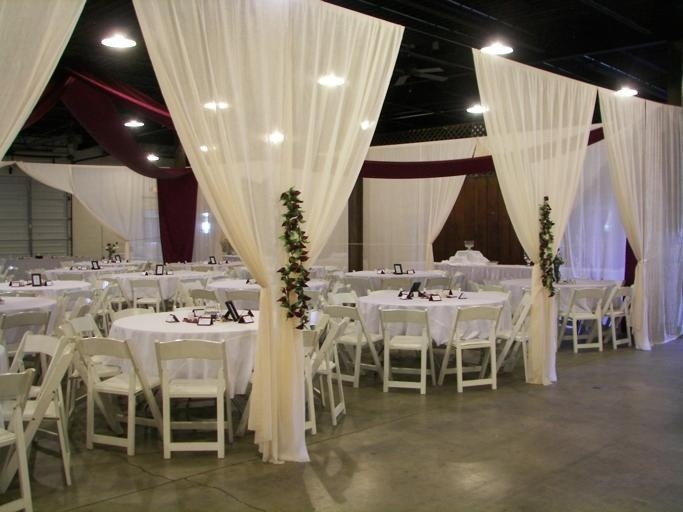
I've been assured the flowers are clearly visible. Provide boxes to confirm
[105,242,119,255]
